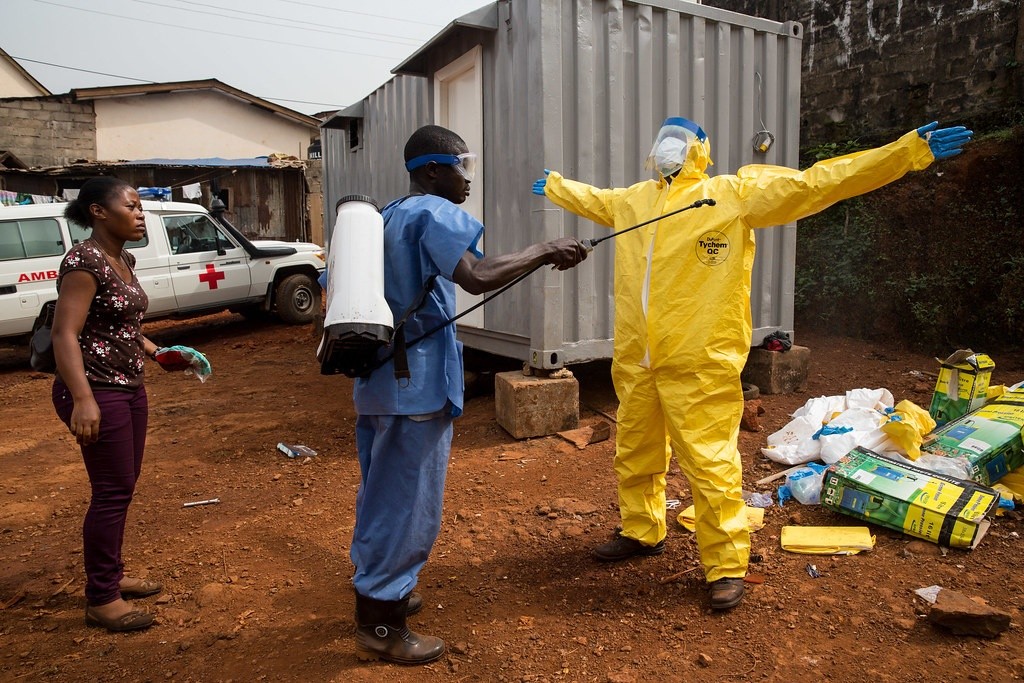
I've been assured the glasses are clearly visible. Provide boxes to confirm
[405,153,476,181]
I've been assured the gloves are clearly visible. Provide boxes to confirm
[917,121,973,159]
[532,169,551,195]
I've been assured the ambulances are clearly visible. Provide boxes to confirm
[0,187,326,338]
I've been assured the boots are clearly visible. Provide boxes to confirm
[355,588,446,663]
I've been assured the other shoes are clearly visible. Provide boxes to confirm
[85,607,153,631]
[120,579,163,598]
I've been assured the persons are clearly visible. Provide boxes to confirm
[52,176,190,633]
[350,124,587,665]
[535,119,974,611]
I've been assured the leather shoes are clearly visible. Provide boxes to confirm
[593,531,665,559]
[711,578,745,608]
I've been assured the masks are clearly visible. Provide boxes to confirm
[655,137,685,176]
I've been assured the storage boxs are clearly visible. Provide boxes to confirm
[929,349,995,429]
[919,387,1024,488]
[819,445,1001,552]
